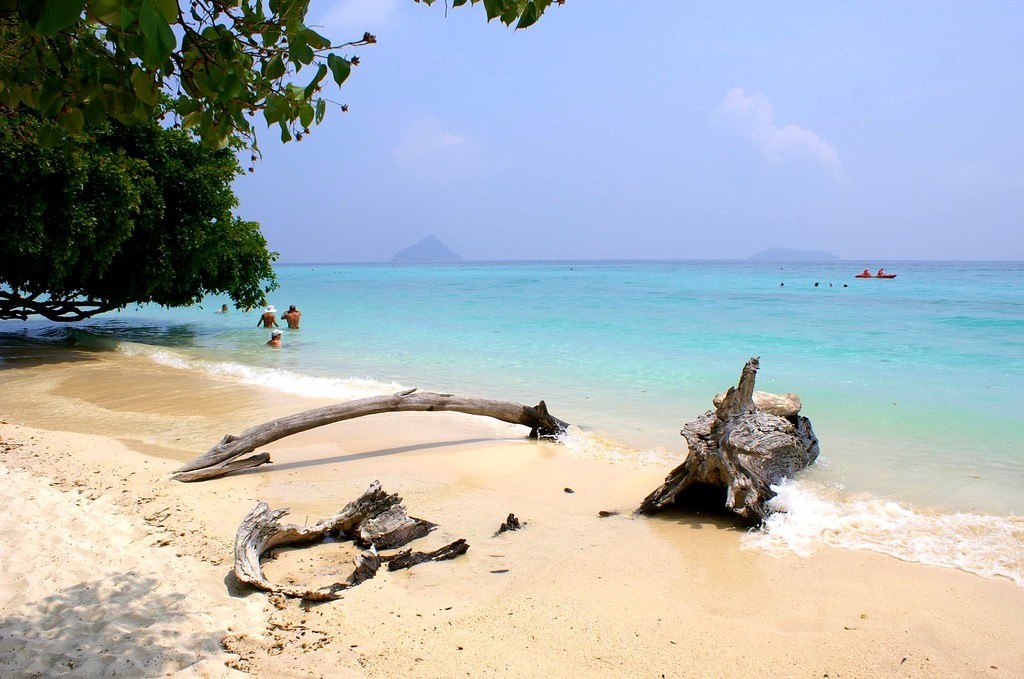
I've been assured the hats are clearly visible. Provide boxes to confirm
[264,305,277,312]
[271,329,283,337]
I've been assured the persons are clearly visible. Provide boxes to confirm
[266,329,283,346]
[215,303,228,313]
[281,305,301,329]
[864,268,872,276]
[256,305,279,329]
[246,307,251,312]
[878,269,886,275]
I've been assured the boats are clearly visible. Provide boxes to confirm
[856,273,898,279]
